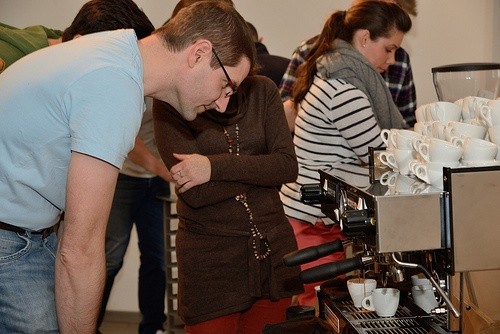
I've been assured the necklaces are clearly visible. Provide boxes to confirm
[221,123,271,261]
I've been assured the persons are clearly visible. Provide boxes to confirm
[0,0,157,75]
[96,97,167,334]
[279,0,412,306]
[245,1,418,139]
[0,0,259,334]
[152,0,305,334]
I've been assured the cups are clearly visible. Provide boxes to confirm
[362,288,400,317]
[347,279,377,307]
[412,273,432,285]
[412,286,442,313]
[379,96,500,191]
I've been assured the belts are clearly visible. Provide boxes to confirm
[0,221,54,235]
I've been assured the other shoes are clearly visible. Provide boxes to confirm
[139,322,167,334]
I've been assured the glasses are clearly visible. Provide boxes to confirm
[193,39,237,98]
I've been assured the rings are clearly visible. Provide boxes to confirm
[176,171,183,178]
